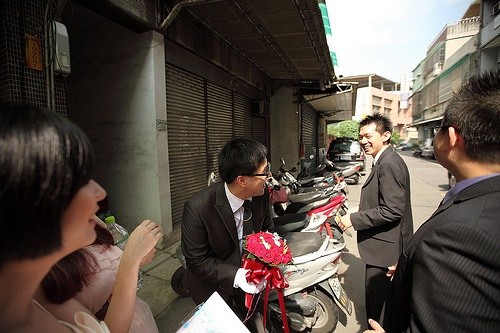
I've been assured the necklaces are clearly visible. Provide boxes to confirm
[234,208,252,221]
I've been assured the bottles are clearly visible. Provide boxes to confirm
[104,216,143,293]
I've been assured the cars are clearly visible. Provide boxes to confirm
[325,136,366,175]
[414,138,437,160]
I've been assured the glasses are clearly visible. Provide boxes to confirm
[434,125,461,136]
[235,161,272,177]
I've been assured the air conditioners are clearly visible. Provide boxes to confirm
[251,99,272,119]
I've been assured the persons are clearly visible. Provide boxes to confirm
[363,69,500,333]
[181,138,275,333]
[337,113,413,333]
[0,100,111,333]
[34,214,164,333]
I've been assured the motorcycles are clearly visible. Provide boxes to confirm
[207,147,363,333]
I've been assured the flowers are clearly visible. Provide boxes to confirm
[241,230,297,269]
